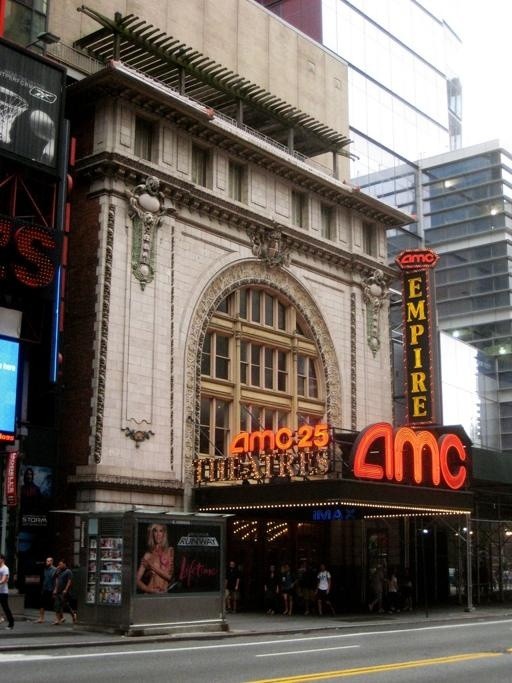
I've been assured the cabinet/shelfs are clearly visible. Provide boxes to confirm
[79,534,128,630]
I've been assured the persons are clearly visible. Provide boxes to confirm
[136,523,176,594]
[20,468,42,501]
[52,558,77,624]
[222,560,337,616]
[33,556,65,623]
[1,553,14,629]
[41,475,52,501]
[367,557,416,615]
